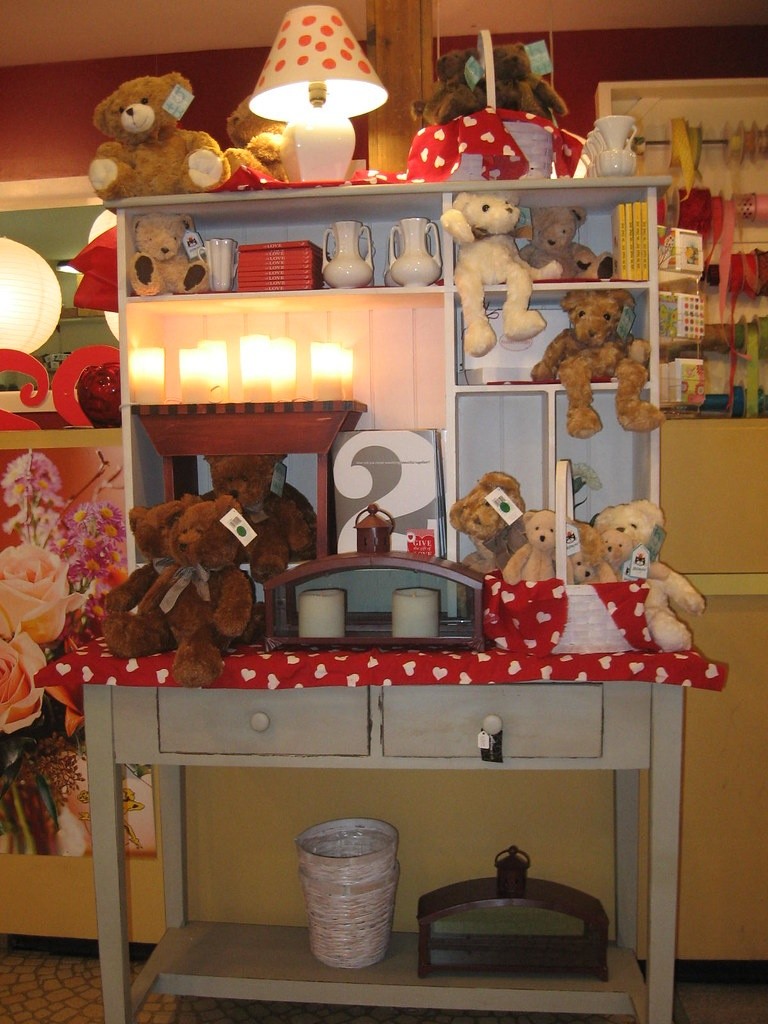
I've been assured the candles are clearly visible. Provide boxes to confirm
[392,586,439,639]
[299,588,347,638]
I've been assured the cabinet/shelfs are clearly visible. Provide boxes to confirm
[34,168,686,1024]
[594,76,768,971]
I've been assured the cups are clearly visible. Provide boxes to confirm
[389,217,443,289]
[578,114,638,178]
[322,220,374,289]
[198,239,238,293]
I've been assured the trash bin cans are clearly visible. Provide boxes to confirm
[296,819,402,972]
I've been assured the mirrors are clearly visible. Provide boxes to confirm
[0,174,120,412]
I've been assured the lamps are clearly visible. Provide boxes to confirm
[246,4,387,183]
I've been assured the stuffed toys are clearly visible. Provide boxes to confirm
[439,191,546,358]
[224,95,289,183]
[104,454,316,687]
[450,470,705,652]
[411,42,568,124]
[531,290,665,439]
[519,206,614,280]
[129,212,210,297]
[86,71,231,216]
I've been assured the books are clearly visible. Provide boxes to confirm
[236,240,331,290]
[609,202,650,282]
